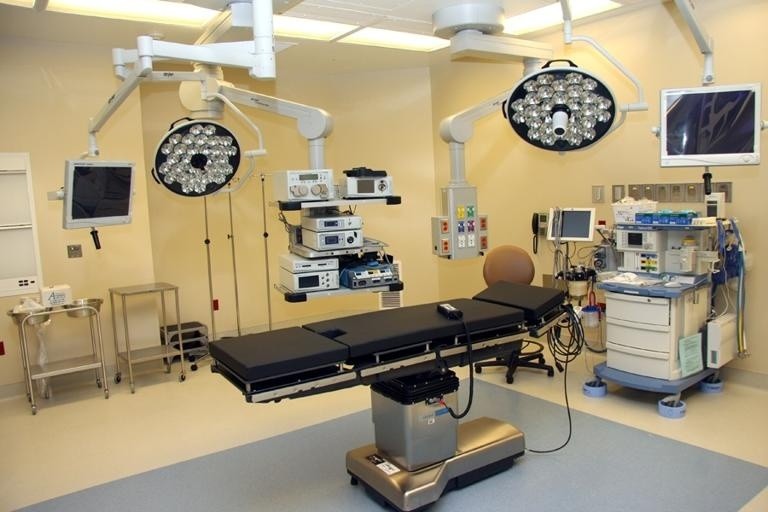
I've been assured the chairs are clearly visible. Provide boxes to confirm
[468,244,554,382]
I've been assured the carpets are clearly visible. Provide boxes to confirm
[23,377,768,512]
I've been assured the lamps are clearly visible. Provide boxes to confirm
[150,91,268,197]
[498,0,650,153]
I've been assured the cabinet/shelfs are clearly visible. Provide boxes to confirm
[158,321,209,364]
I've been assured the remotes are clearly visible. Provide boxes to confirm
[437,303,464,320]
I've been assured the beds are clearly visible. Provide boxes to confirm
[207,280,572,403]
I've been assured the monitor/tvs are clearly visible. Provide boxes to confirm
[62,160,135,229]
[659,83,761,168]
[546,207,596,243]
